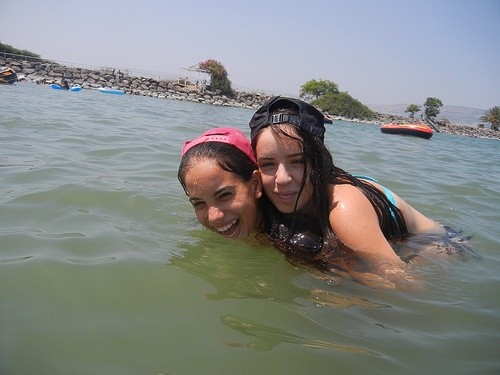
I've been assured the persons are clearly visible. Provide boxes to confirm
[248,96,434,262]
[177,127,264,241]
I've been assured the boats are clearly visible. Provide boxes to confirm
[379,121,435,140]
[48,83,82,92]
[96,87,126,96]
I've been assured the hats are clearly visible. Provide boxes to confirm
[248,95,326,145]
[181,127,257,164]
